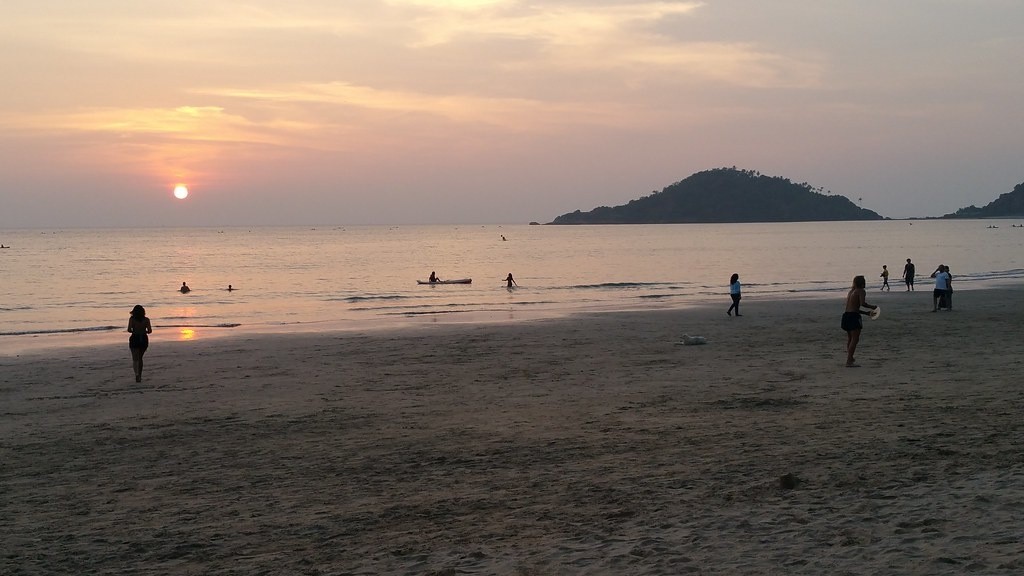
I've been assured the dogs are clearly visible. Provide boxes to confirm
[673,333,708,345]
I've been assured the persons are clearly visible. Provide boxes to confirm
[502,273,518,287]
[930,264,954,313]
[902,259,916,292]
[181,282,190,291]
[501,235,506,241]
[429,271,441,282]
[228,285,232,289]
[880,265,891,291]
[841,275,878,367]
[128,305,152,382]
[727,273,742,316]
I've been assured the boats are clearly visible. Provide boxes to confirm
[1012,224,1024,227]
[986,225,998,228]
[415,278,473,285]
